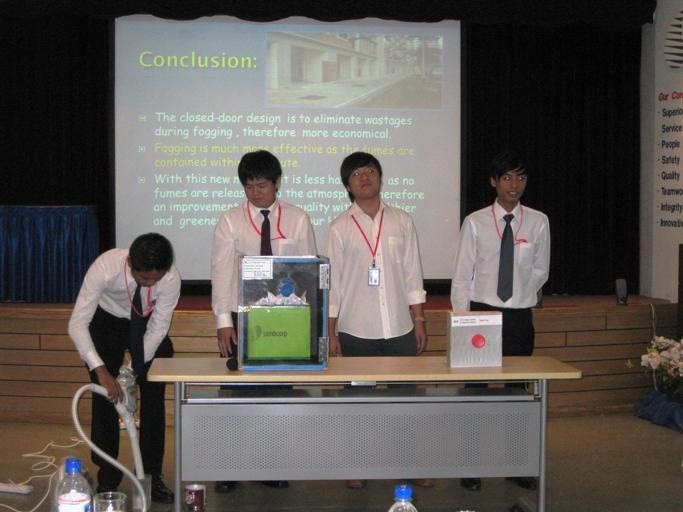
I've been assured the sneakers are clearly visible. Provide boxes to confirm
[345,480,366,489]
[410,479,433,487]
[460,478,481,491]
[506,477,537,490]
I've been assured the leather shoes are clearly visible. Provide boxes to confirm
[96,480,117,511]
[134,472,174,503]
[250,481,289,489]
[215,481,235,493]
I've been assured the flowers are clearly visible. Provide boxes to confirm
[640,333,682,400]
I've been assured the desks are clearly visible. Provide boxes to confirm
[140,353,581,511]
[0,197,102,309]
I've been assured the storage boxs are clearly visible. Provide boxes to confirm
[235,252,332,373]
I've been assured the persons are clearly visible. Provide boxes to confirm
[210,149,319,493]
[66,232,174,505]
[449,151,551,491]
[323,151,435,489]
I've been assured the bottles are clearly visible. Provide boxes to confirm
[387,484,419,511]
[55,454,93,512]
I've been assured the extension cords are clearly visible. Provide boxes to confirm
[1,481,35,495]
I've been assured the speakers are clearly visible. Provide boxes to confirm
[614,278,628,306]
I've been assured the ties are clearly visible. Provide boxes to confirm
[260,210,272,255]
[496,214,514,302]
[128,285,148,387]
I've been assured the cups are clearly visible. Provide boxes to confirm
[185,484,207,512]
[94,491,128,512]
